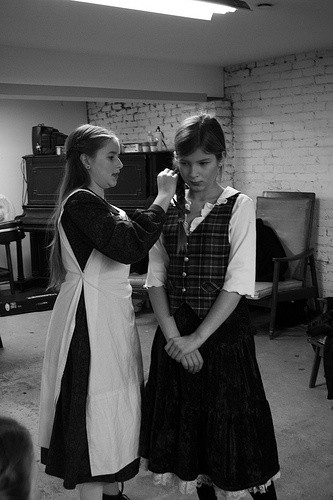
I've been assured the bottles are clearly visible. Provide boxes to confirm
[153,129,162,152]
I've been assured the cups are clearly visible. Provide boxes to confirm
[150,142,157,152]
[55,146,66,155]
[142,142,150,152]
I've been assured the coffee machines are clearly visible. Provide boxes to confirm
[31,124,67,155]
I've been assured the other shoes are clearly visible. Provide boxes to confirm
[102,490,131,500]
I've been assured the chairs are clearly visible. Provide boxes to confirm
[240,191,319,340]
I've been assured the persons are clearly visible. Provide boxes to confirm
[0,416,37,500]
[138,115,282,500]
[38,123,179,500]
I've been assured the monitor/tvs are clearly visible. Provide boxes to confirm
[31,125,68,155]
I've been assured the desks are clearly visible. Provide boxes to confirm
[0,224,27,297]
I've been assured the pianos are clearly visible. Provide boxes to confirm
[14,151,185,308]
